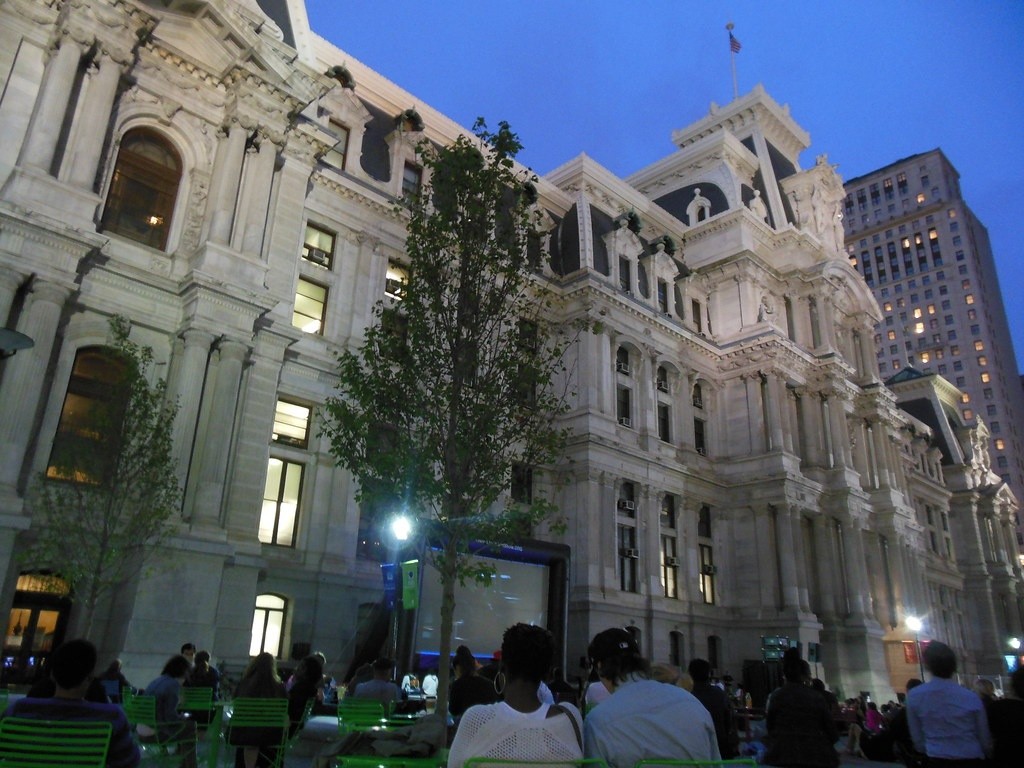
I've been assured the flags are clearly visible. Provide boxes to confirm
[729,30,742,54]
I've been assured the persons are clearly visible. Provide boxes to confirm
[449,645,590,716]
[584,626,752,768]
[84,659,144,705]
[285,650,440,741]
[764,647,839,768]
[904,639,993,768]
[224,652,286,768]
[448,623,586,768]
[309,695,457,768]
[136,655,198,768]
[1,639,142,768]
[974,667,1024,768]
[843,678,928,768]
[177,642,222,744]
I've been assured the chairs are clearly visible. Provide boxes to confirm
[0,686,761,768]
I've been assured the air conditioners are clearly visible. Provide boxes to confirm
[706,566,718,574]
[617,363,629,373]
[619,417,631,427]
[669,557,681,566]
[664,313,672,319]
[626,548,640,558]
[308,249,326,263]
[625,291,634,298]
[386,279,399,293]
[404,197,414,207]
[622,500,635,510]
[699,332,706,338]
[697,447,707,456]
[658,381,669,391]
[694,397,703,407]
[523,338,535,348]
[462,377,473,388]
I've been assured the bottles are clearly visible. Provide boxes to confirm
[745,692,752,709]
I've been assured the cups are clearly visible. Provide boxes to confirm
[426,696,436,714]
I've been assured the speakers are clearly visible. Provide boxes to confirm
[580,656,590,670]
[292,642,310,660]
